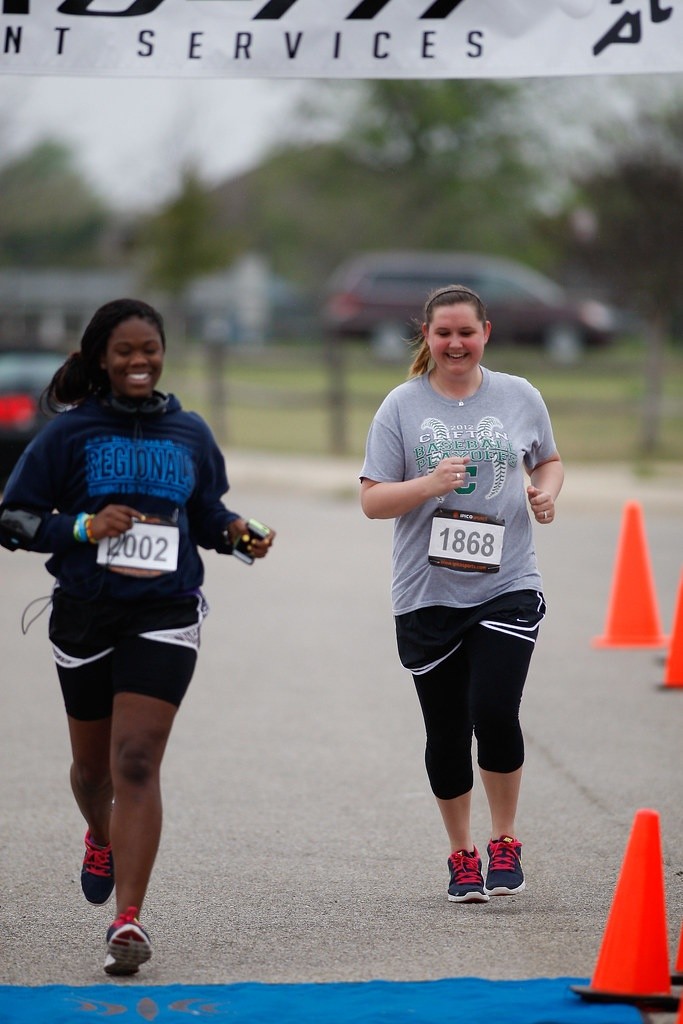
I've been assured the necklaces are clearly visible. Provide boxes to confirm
[435,376,464,406]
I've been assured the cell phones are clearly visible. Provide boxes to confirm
[231,519,269,565]
[0,508,43,539]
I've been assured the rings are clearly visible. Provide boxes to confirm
[457,473,460,480]
[544,512,547,519]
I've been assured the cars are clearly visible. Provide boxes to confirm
[1,344,86,488]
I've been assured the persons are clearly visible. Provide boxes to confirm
[360,285,565,902]
[0,299,274,976]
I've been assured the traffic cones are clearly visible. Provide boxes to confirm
[593,500,673,651]
[568,808,678,1006]
[657,563,683,691]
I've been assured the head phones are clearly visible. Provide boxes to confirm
[96,384,170,417]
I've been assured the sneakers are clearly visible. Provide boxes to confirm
[447,845,490,902]
[81,831,116,906]
[484,835,525,895]
[103,907,152,975]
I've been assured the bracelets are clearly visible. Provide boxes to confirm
[86,518,98,543]
[74,512,90,542]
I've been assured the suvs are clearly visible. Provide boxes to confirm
[323,249,624,368]
